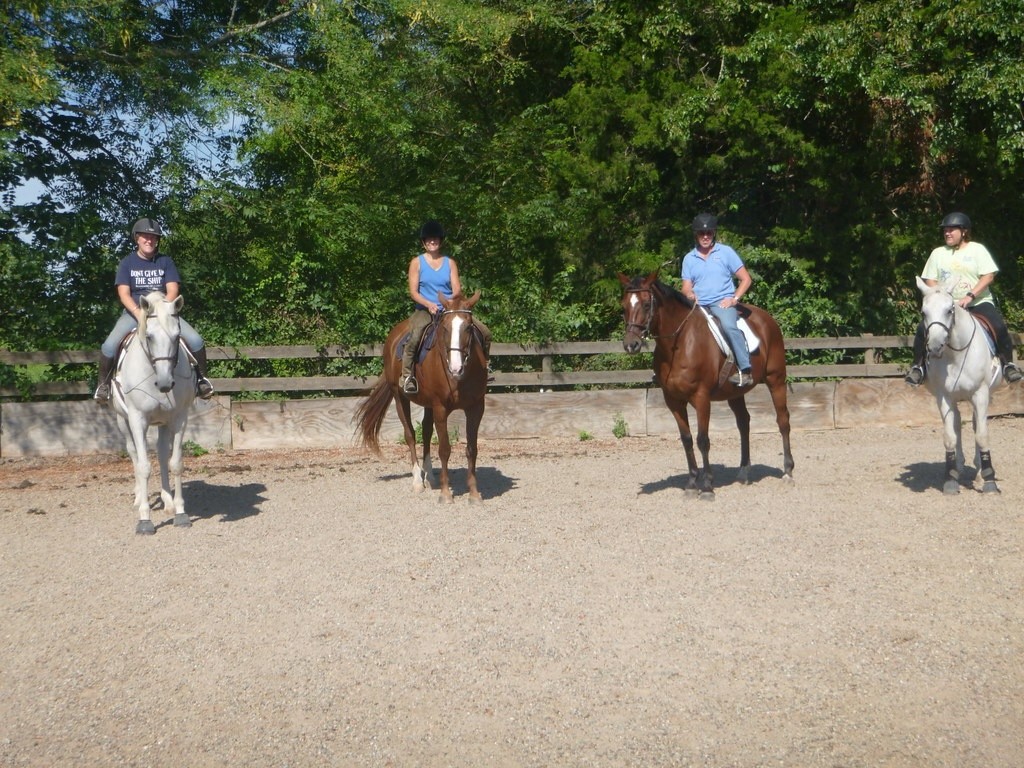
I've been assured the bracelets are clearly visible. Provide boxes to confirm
[733,296,740,302]
[132,306,138,315]
[967,292,975,299]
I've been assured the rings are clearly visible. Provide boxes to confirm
[432,311,433,313]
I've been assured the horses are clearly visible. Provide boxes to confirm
[915,274,1003,496]
[352,288,488,506]
[107,291,199,535]
[615,265,795,502]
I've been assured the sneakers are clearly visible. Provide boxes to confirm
[1005,367,1022,382]
[652,374,658,385]
[728,369,754,385]
[487,375,495,381]
[906,368,925,385]
[399,376,417,391]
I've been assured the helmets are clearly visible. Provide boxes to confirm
[692,213,717,230]
[420,221,444,238]
[939,212,971,229]
[132,218,162,237]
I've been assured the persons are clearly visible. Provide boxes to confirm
[93,218,212,404]
[399,222,461,391]
[906,213,1022,383]
[681,214,753,384]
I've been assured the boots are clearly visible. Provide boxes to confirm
[97,350,114,409]
[192,344,212,399]
